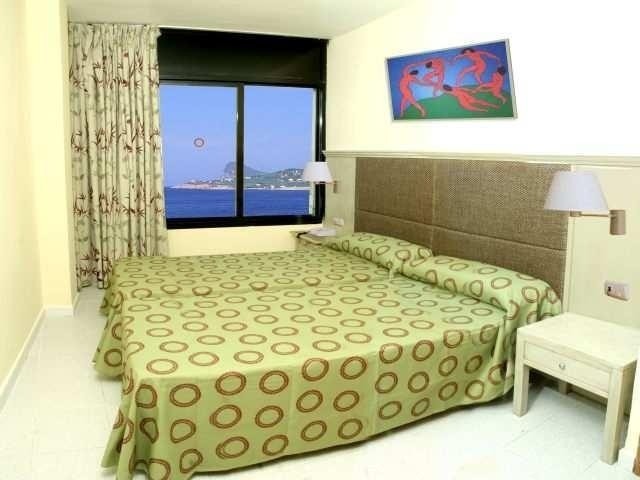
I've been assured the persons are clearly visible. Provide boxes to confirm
[451,47,502,87]
[471,61,510,105]
[417,58,453,99]
[427,78,501,114]
[397,59,439,118]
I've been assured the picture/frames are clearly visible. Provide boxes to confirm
[385,38,517,124]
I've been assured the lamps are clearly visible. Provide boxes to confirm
[543,169,626,236]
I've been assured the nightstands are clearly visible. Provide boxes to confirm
[512,311,639,464]
